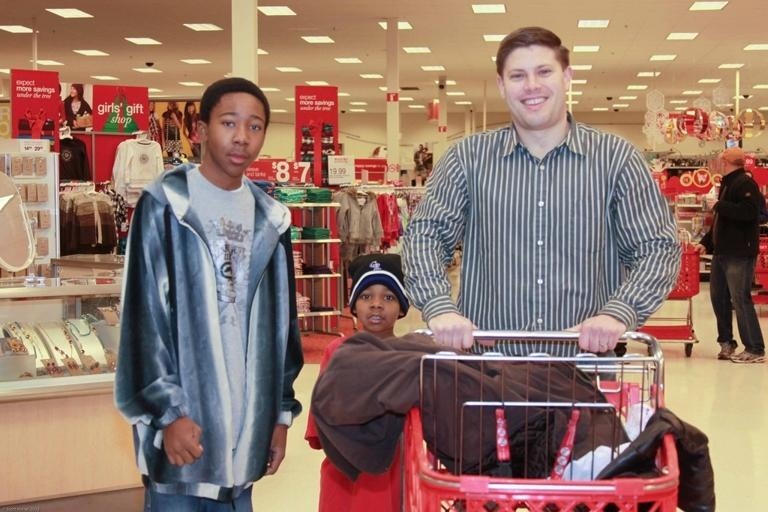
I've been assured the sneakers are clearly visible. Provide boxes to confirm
[729,349,766,364]
[717,342,735,360]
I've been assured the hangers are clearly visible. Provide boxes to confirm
[58,126,73,140]
[57,181,116,199]
[132,130,152,145]
[340,183,427,206]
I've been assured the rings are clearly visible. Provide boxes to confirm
[597,343,608,348]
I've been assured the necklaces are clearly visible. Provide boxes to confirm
[0,315,116,381]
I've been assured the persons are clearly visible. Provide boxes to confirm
[413,142,432,180]
[303,252,413,510]
[160,100,182,159]
[63,85,92,132]
[181,101,203,159]
[113,77,305,510]
[147,102,160,144]
[399,25,685,380]
[693,148,767,363]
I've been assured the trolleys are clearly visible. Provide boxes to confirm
[402,326,679,512]
[636,229,701,356]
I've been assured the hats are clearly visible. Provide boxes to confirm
[347,259,409,320]
[716,148,745,165]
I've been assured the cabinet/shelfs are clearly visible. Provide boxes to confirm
[271,201,341,333]
[666,154,768,179]
[674,191,718,275]
